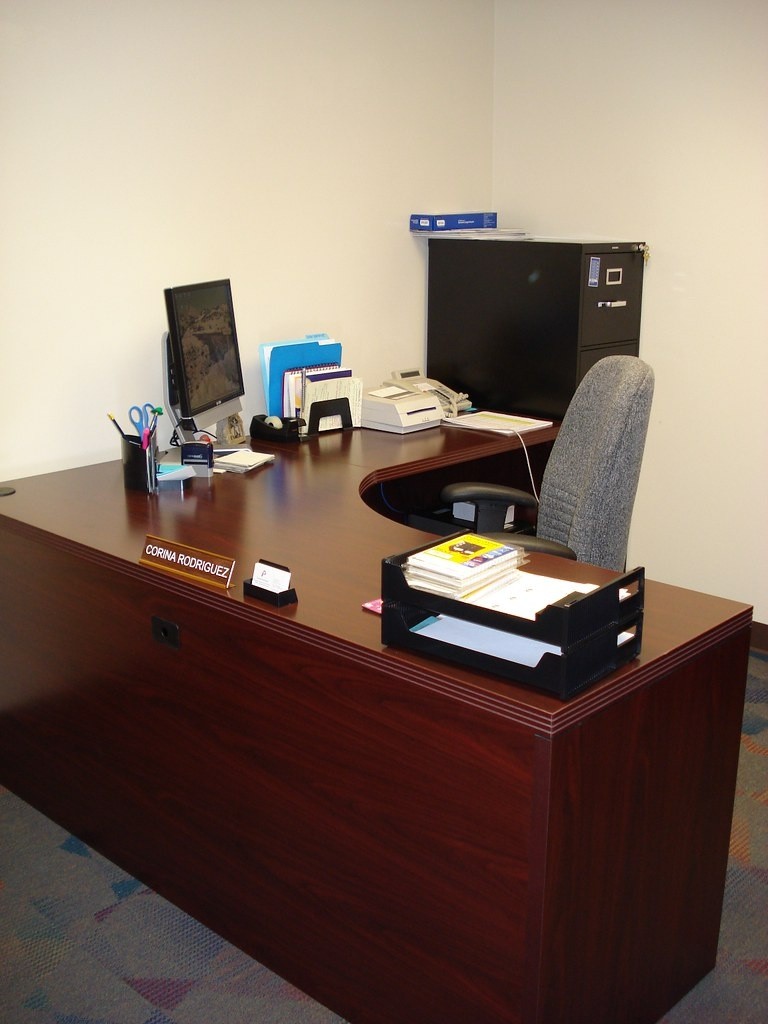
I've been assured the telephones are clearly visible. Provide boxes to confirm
[380,367,473,413]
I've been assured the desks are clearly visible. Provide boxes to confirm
[0,404,753,1024]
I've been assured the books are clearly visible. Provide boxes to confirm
[283,361,363,434]
[214,450,274,473]
[403,532,522,603]
[441,410,553,437]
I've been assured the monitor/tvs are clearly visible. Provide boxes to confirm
[160,277,254,455]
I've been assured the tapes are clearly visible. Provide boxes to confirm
[264,415,283,429]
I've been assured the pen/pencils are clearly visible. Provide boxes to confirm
[107,412,126,440]
[139,406,163,449]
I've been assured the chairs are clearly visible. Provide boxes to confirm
[444,355,655,574]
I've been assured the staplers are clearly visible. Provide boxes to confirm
[280,417,311,443]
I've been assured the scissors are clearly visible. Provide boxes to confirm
[128,403,156,443]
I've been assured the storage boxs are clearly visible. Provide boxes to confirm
[410,212,497,231]
